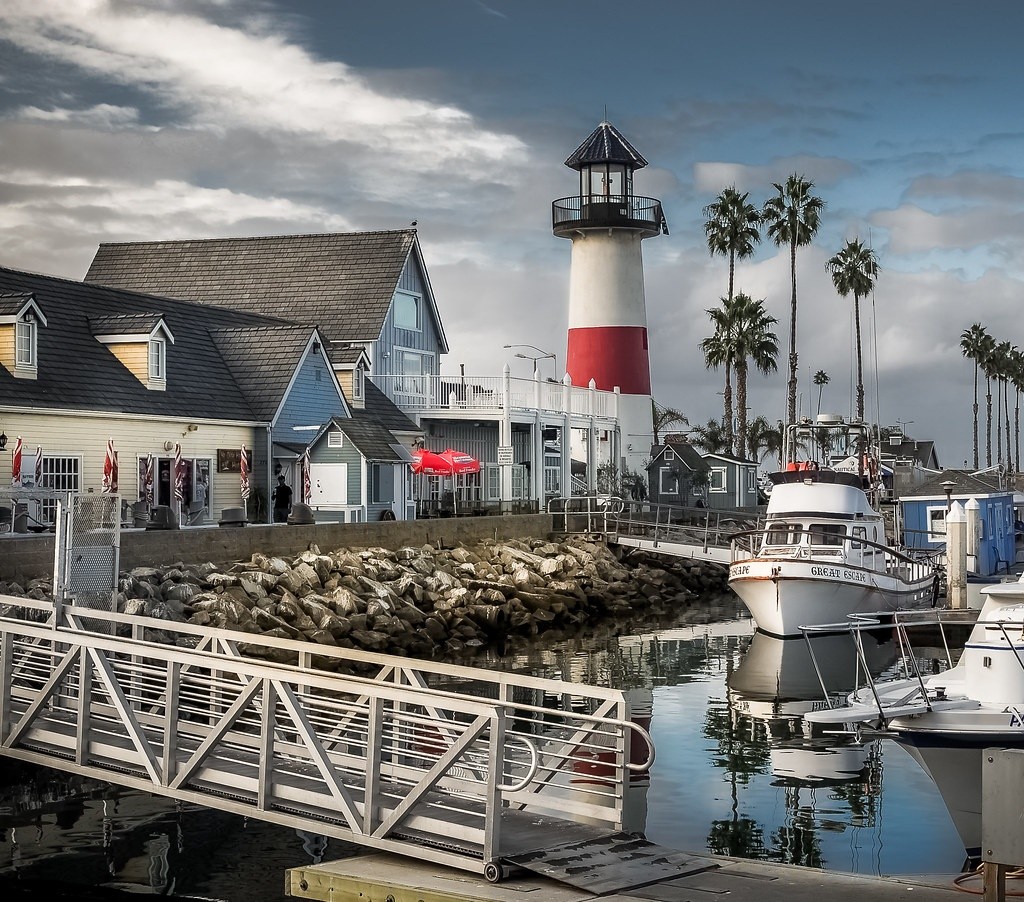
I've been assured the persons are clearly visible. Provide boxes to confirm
[696,497,706,508]
[139,491,145,502]
[272,475,292,523]
[631,478,647,512]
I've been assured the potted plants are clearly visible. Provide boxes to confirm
[440,491,454,518]
[247,485,268,525]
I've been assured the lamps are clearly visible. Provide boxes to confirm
[382,351,391,359]
[275,462,283,475]
[0,430,8,451]
[164,440,173,451]
[314,341,321,355]
[24,308,34,322]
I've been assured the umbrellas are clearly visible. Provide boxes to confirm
[145,452,154,519]
[11,436,23,533]
[101,436,113,528]
[305,447,312,504]
[240,445,250,519]
[111,451,118,523]
[173,444,183,529]
[34,446,42,525]
[409,450,480,515]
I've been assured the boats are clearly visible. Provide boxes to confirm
[730,412,943,638]
[729,638,894,833]
[891,734,1024,873]
[805,585,1024,732]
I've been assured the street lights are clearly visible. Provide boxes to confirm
[503,345,557,381]
